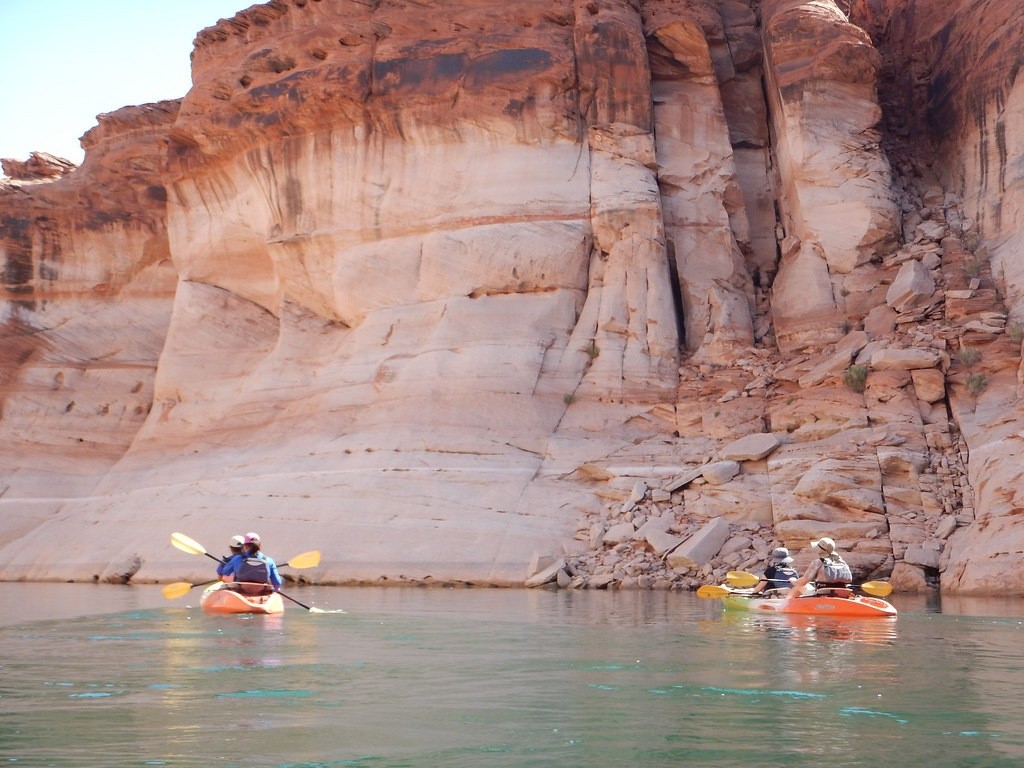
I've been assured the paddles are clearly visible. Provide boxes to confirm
[726,570,893,597]
[170,532,326,614]
[161,549,321,601]
[695,584,762,600]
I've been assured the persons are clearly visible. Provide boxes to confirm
[216,532,282,594]
[753,547,799,593]
[788,537,853,598]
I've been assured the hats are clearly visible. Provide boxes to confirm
[810,537,835,558]
[771,547,794,563]
[229,535,245,547]
[244,533,261,550]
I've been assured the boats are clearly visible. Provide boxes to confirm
[199,580,286,614]
[697,583,817,602]
[720,587,898,620]
